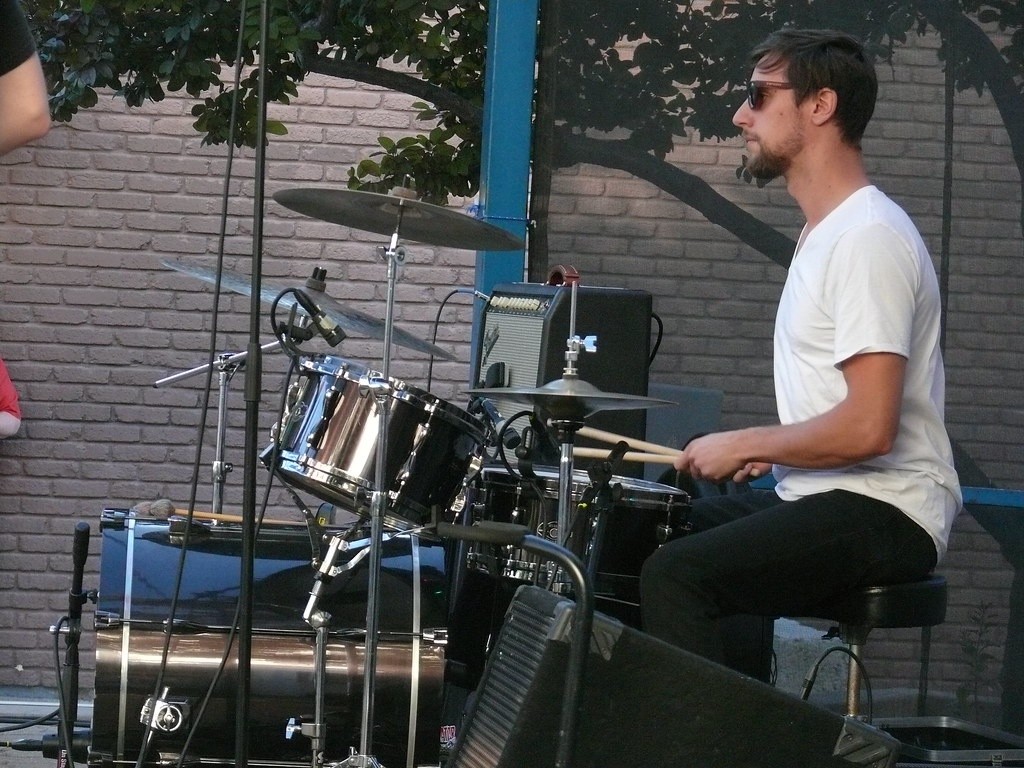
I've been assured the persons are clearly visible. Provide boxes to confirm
[0,357,21,440]
[638,27,963,669]
[0,0,50,157]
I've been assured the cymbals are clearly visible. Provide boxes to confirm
[270,185,526,252]
[462,380,679,416]
[157,254,458,364]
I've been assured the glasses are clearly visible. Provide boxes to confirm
[746,80,802,111]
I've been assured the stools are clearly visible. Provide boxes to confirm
[807,577,948,718]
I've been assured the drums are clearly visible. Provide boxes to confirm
[265,358,487,536]
[460,460,696,610]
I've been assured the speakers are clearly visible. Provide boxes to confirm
[443,583,904,768]
[469,283,653,480]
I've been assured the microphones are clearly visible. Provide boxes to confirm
[12,730,90,764]
[527,412,562,458]
[481,399,522,449]
[292,289,348,347]
[569,441,630,528]
